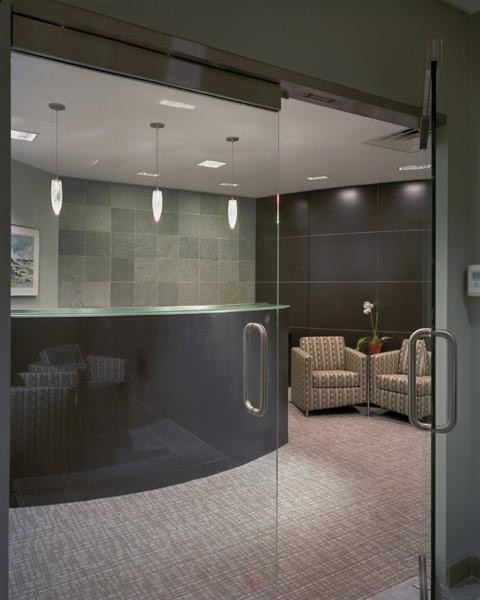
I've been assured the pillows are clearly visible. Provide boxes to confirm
[299,336,345,370]
[399,339,428,376]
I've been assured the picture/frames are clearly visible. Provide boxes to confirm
[11,224,40,297]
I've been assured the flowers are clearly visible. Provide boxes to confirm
[356,300,393,351]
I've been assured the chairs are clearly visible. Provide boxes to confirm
[291,336,369,417]
[370,349,432,420]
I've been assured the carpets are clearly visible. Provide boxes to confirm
[9,386,431,600]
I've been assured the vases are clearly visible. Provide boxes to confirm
[368,343,382,354]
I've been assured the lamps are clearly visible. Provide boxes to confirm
[151,123,165,221]
[48,103,66,216]
[225,137,239,229]
